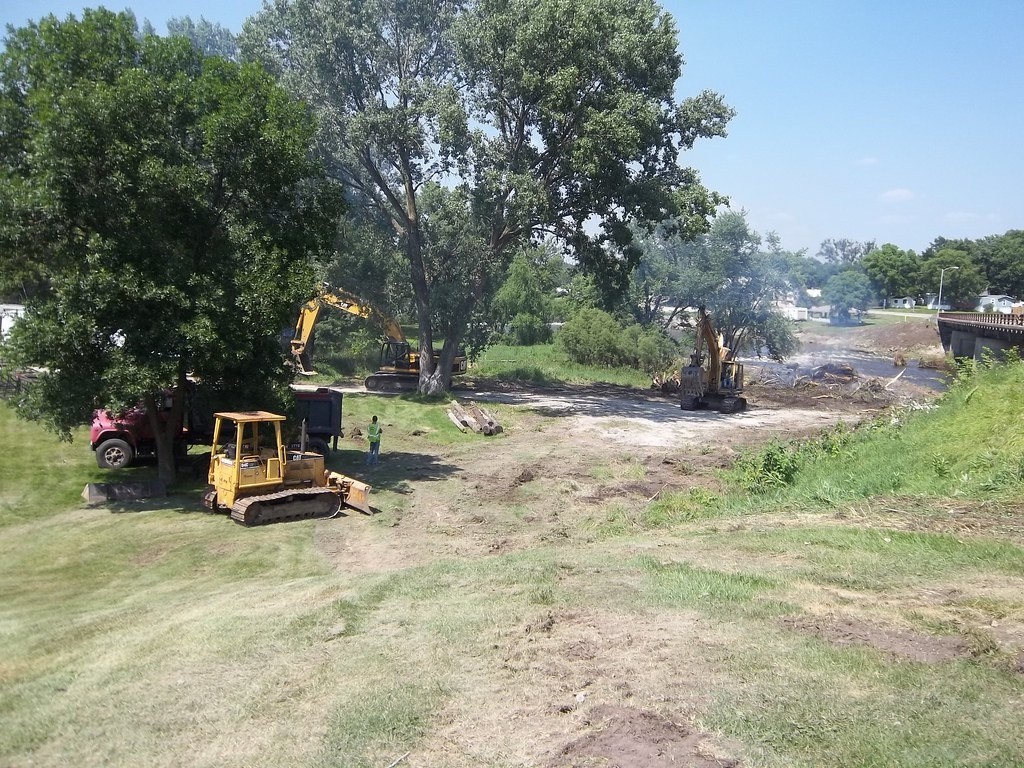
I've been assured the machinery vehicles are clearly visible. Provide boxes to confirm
[679,307,748,413]
[288,281,468,393]
[203,408,377,525]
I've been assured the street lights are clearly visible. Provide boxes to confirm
[935,267,960,318]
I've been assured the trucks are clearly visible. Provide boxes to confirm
[87,379,343,470]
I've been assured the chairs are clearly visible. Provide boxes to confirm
[227,444,235,459]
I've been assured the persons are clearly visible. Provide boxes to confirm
[723,369,732,388]
[367,416,382,465]
